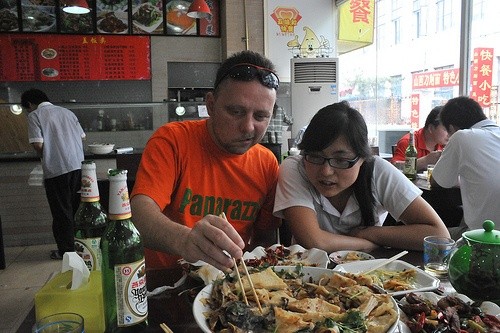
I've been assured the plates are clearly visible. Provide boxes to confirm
[333,259,440,297]
[9,12,56,31]
[167,1,194,32]
[132,3,163,32]
[41,68,58,77]
[96,15,128,34]
[192,266,400,333]
[329,250,374,264]
[397,292,500,333]
[41,48,57,60]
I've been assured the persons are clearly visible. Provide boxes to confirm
[129,50,279,273]
[390,106,450,172]
[273,100,452,254]
[430,96,500,242]
[21,89,86,260]
[259,101,294,143]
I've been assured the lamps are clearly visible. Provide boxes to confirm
[62,0,90,14]
[186,0,212,19]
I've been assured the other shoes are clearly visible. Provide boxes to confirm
[49,250,64,259]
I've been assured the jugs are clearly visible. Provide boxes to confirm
[447,220,500,306]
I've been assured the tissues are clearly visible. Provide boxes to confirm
[34,251,106,333]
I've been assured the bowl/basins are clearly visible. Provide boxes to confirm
[88,144,115,154]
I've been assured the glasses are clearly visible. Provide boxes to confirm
[304,147,360,169]
[213,63,279,97]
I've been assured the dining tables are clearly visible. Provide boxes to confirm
[15,247,500,333]
[412,179,462,208]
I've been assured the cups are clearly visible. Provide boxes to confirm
[423,236,455,280]
[427,164,433,187]
[32,312,85,333]
[242,244,329,269]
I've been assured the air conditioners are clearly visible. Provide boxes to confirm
[290,58,339,145]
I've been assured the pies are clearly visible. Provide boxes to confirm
[234,267,399,333]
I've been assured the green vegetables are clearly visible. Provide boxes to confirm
[63,16,86,34]
[139,0,160,27]
[211,260,368,333]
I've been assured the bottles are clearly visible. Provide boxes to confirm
[403,132,417,181]
[73,160,108,271]
[97,110,105,131]
[99,170,149,333]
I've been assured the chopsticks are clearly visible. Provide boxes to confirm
[219,212,264,314]
[160,322,173,333]
[359,251,409,276]
